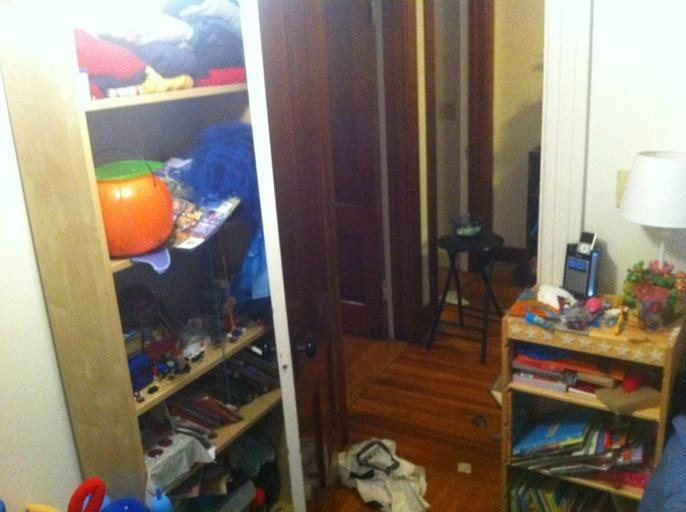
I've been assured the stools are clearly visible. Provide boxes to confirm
[427,233,504,364]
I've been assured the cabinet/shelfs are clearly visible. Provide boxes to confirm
[500,283,686,511]
[0,0,296,512]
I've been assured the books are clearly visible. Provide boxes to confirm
[503,337,643,512]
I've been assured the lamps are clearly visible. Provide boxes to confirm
[618,149,686,330]
